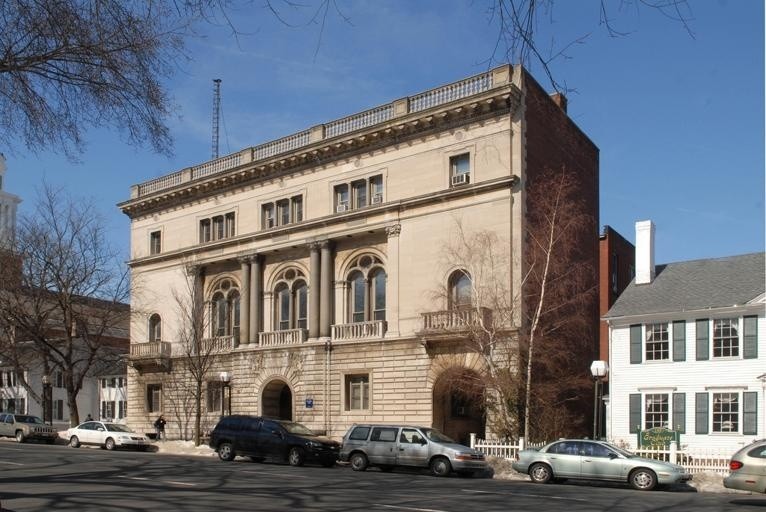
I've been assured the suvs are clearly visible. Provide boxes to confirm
[0,412,59,444]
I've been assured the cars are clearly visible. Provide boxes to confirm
[65,420,151,451]
[722,437,766,494]
[511,438,694,491]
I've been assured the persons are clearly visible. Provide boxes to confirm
[153,414,167,441]
[84,413,93,422]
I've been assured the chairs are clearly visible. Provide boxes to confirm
[394,432,420,443]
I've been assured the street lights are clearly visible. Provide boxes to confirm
[41,374,53,426]
[589,359,608,441]
[220,372,232,419]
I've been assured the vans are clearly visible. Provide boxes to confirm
[337,422,489,479]
[207,413,341,468]
